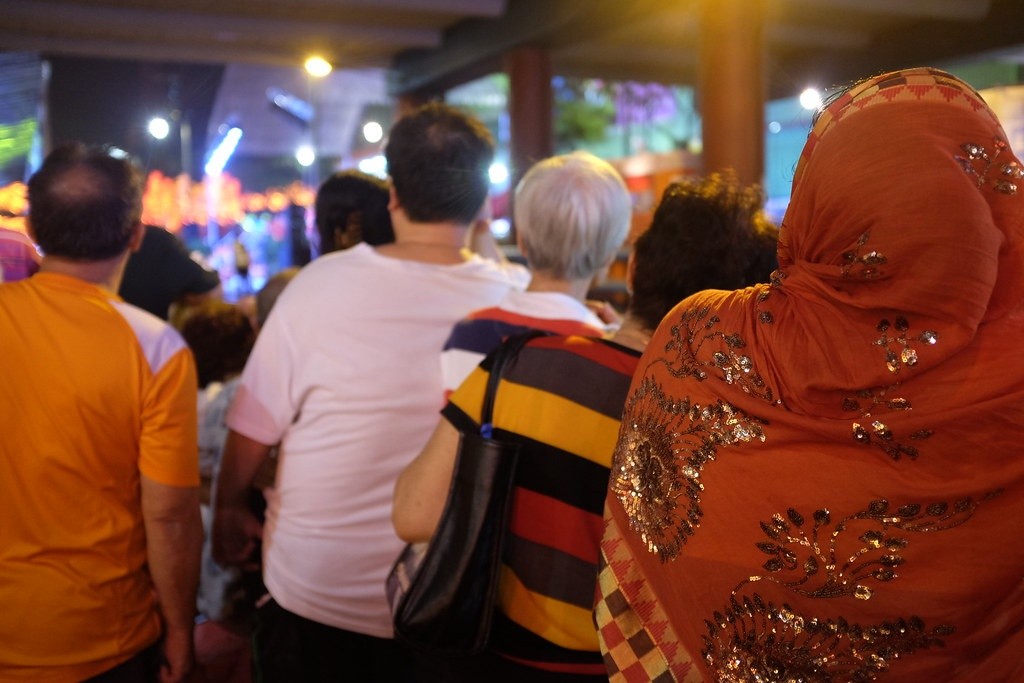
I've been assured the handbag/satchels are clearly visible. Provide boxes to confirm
[383,331,546,655]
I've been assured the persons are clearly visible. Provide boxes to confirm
[0,145,205,683]
[3,172,395,683]
[210,108,536,682]
[442,156,634,408]
[391,174,782,683]
[601,66,1024,683]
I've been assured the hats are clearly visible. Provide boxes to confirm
[120,223,219,300]
